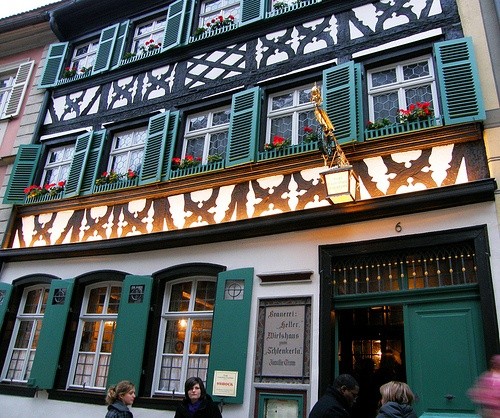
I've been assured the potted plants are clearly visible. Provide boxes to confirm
[208,153,222,169]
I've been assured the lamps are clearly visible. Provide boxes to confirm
[319,166,359,206]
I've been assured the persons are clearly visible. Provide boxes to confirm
[174,377,222,418]
[105,380,136,418]
[308,347,419,418]
[468,355,500,418]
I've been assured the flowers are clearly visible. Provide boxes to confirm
[127,169,138,179]
[303,126,318,143]
[23,184,42,198]
[183,156,202,168]
[207,15,234,30]
[95,170,119,185]
[264,136,291,152]
[368,118,392,129]
[42,181,65,195]
[396,101,432,126]
[171,157,180,170]
[80,66,93,73]
[140,39,162,52]
[61,67,77,78]
